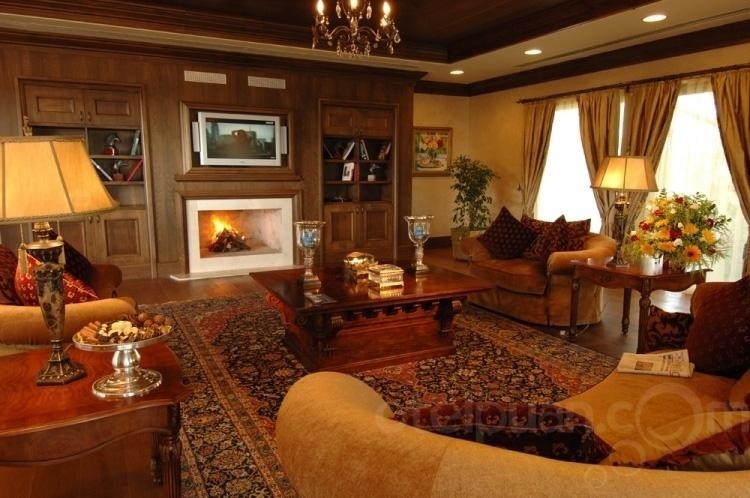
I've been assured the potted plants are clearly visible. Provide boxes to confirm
[450,159,503,263]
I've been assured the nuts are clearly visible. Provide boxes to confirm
[136,312,167,328]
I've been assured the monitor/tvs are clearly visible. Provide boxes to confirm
[197,111,281,167]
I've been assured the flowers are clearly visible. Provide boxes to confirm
[616,188,734,281]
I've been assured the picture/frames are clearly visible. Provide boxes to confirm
[412,126,453,176]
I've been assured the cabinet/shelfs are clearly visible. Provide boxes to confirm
[0,343,196,498]
[319,99,398,266]
[12,75,156,281]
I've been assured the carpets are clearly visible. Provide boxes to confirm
[139,289,620,498]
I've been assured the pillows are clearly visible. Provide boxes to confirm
[50,229,93,288]
[13,248,99,306]
[519,212,591,264]
[388,405,616,466]
[522,214,585,272]
[601,411,750,472]
[476,206,538,260]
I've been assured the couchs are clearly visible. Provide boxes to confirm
[275,282,750,497]
[459,205,619,328]
[2,245,136,354]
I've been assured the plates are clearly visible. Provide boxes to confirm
[72,324,175,399]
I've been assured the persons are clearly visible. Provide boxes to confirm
[232,130,252,151]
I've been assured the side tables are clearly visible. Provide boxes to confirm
[568,260,714,355]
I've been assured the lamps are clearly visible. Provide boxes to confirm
[311,0,401,58]
[589,155,660,268]
[1,136,117,386]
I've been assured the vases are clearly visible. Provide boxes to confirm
[663,250,686,273]
